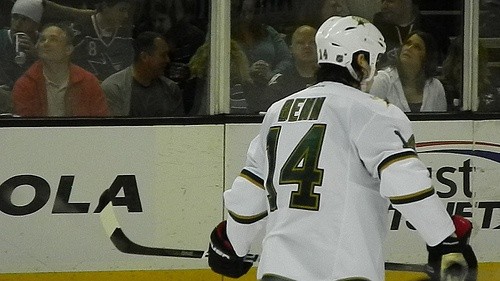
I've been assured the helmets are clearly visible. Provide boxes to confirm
[315,14,386,66]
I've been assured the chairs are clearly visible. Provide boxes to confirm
[0,0,500,119]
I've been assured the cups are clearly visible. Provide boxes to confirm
[12,32,28,64]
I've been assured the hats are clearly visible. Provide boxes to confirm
[11,0,44,23]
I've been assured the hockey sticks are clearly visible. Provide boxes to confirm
[98,188,429,274]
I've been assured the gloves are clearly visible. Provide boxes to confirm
[426,239,478,280]
[207,220,259,278]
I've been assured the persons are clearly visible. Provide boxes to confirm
[208,15,477,281]
[365,0,500,114]
[0,0,319,120]
